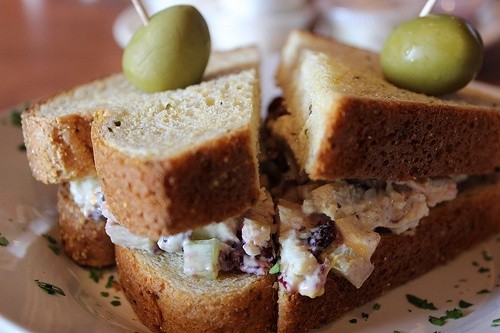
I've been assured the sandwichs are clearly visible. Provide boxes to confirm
[21,42,280,333]
[259,29,500,333]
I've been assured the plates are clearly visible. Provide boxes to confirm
[0,44,499,333]
